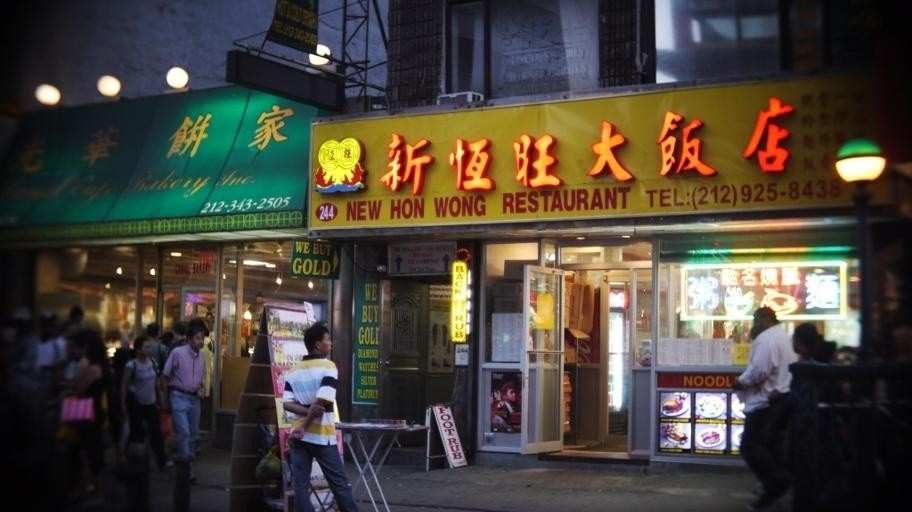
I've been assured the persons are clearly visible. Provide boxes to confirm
[496,382,518,420]
[768,324,845,512]
[732,307,798,512]
[282,327,362,512]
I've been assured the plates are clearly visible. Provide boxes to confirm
[659,392,751,451]
[760,293,800,316]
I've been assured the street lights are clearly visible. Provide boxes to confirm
[834,136,888,511]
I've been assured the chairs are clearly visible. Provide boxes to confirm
[283,448,336,511]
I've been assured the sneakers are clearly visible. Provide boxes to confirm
[160,447,200,483]
[745,483,790,511]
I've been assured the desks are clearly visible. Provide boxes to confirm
[333,422,432,512]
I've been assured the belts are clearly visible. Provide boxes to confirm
[173,387,198,396]
